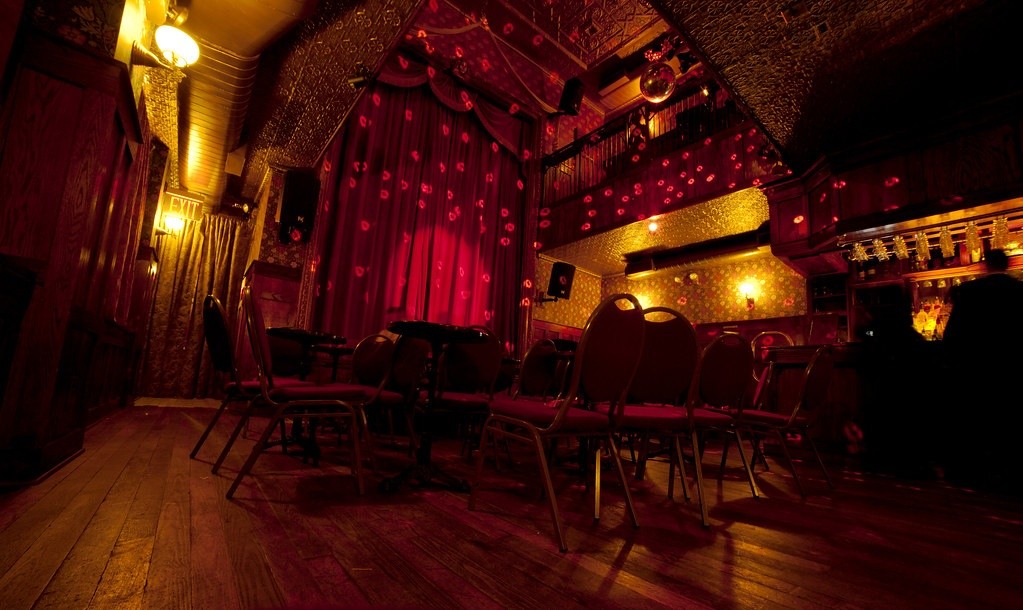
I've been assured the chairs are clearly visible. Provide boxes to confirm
[186,294,835,553]
[677,88,720,147]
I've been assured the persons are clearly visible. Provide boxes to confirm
[943,250,1023,345]
[628,115,649,146]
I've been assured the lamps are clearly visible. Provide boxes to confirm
[131,25,200,74]
[700,79,720,97]
[349,70,381,93]
[243,203,249,220]
[155,213,185,236]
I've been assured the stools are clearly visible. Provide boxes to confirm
[312,345,354,447]
[379,322,486,494]
[268,327,346,467]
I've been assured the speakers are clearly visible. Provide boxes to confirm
[547,262,576,299]
[558,76,585,115]
[278,169,321,245]
